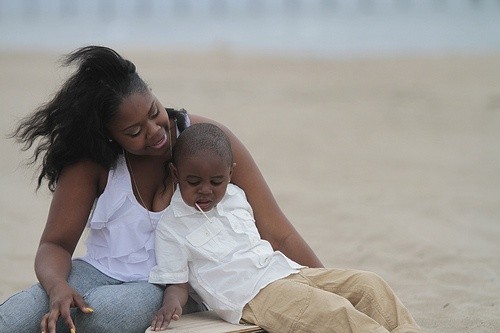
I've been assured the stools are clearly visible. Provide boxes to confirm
[144,311,265,333]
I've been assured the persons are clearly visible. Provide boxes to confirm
[148,123,420,332]
[1,47,327,333]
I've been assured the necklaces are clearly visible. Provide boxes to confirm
[124,127,175,212]
